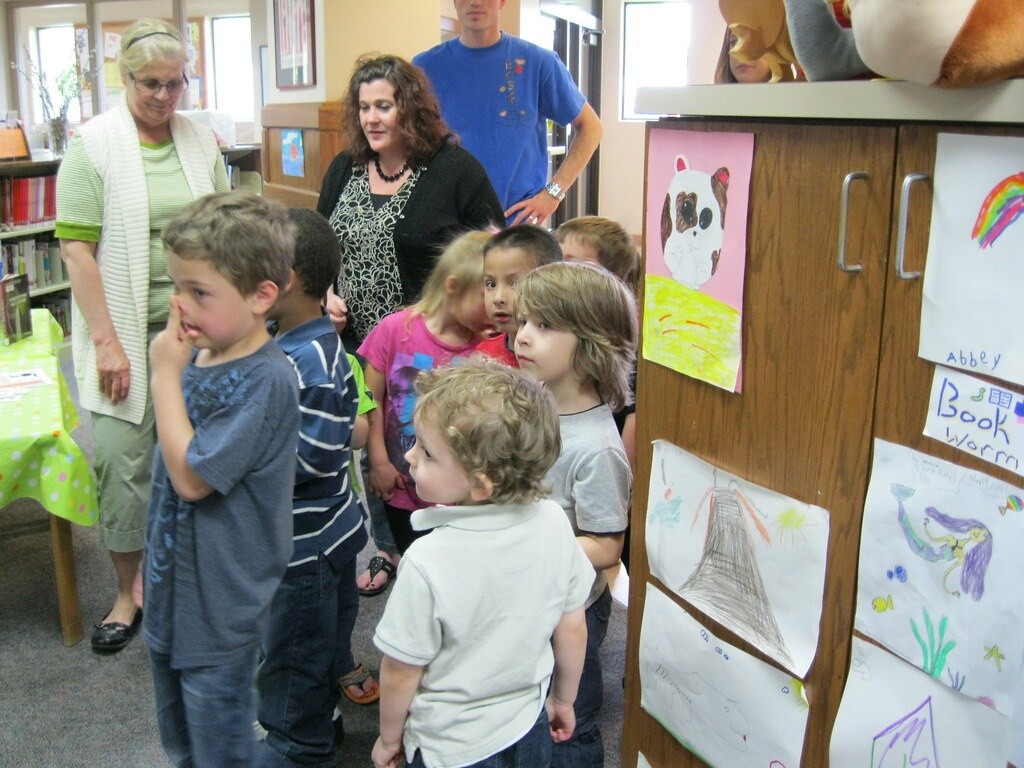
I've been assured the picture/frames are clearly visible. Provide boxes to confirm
[273,0,316,89]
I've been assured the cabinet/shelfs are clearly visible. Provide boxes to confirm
[618,78,1024,768]
[0,146,263,352]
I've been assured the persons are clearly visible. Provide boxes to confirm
[336,220,639,704]
[317,56,508,597]
[55,19,233,652]
[714,25,772,84]
[253,207,368,767]
[141,191,300,768]
[411,1,605,226]
[371,358,596,767]
[514,262,638,768]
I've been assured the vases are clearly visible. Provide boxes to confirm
[48,120,68,156]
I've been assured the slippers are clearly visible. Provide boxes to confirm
[356,555,398,596]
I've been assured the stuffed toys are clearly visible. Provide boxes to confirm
[784,0,1024,89]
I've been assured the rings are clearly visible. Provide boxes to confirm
[529,216,535,220]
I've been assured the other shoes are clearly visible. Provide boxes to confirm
[91,604,142,650]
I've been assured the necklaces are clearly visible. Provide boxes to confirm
[375,153,408,181]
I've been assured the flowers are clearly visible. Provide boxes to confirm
[9,24,107,155]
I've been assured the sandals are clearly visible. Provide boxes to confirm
[334,660,381,704]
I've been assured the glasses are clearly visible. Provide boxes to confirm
[127,68,190,94]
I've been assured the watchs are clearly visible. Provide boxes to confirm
[545,182,566,201]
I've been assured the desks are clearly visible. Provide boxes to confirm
[0,308,99,647]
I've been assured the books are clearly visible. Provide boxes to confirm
[0,273,32,346]
[0,175,58,232]
[0,237,70,292]
[32,297,72,336]
